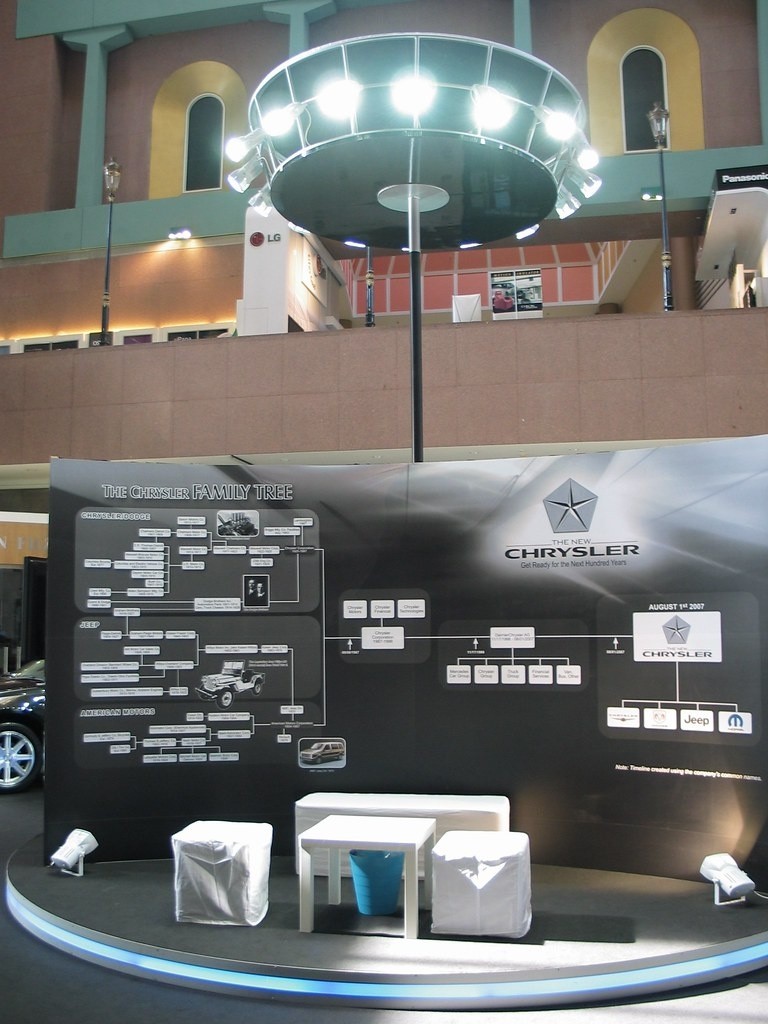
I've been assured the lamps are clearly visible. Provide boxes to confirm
[701,853,756,905]
[640,186,663,202]
[168,226,192,240]
[223,77,604,253]
[47,829,99,877]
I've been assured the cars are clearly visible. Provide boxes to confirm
[0,659,46,797]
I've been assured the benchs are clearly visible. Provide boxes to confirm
[294,793,510,876]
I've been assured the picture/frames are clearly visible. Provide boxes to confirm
[17,333,85,353]
[160,320,235,342]
[115,328,159,346]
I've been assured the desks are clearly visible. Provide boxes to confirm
[297,815,436,939]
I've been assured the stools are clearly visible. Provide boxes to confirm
[170,820,273,926]
[430,830,534,938]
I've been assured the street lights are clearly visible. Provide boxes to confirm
[645,98,678,311]
[98,155,123,347]
